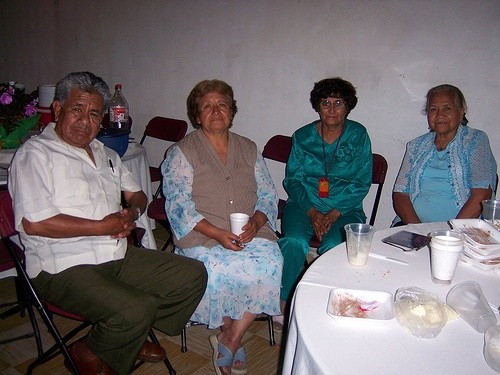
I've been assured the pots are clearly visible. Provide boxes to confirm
[98,127,131,159]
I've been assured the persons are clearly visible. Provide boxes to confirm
[390,84,497,228]
[272,78,373,331]
[8,71,209,375]
[160,79,284,375]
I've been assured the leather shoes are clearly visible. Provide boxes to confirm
[64,343,121,375]
[138,339,167,363]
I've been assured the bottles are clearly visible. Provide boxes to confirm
[109,84,130,129]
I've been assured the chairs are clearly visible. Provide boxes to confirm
[1,116,388,375]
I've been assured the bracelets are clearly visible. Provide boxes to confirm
[133,205,142,220]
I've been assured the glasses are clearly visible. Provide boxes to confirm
[316,99,347,110]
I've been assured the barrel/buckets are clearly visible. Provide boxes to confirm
[38,109,55,132]
[38,84,56,108]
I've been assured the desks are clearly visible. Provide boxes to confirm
[281,221,500,375]
[1,142,156,279]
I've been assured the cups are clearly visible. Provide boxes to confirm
[483,325,500,373]
[344,223,376,266]
[482,199,500,232]
[229,213,249,237]
[430,230,464,285]
[446,281,497,333]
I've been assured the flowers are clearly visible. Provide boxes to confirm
[0,81,38,117]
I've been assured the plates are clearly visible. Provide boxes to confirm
[450,212,500,271]
[327,288,395,326]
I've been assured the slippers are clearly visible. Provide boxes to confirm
[209,334,234,375]
[231,345,248,375]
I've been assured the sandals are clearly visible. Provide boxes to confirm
[273,315,284,333]
